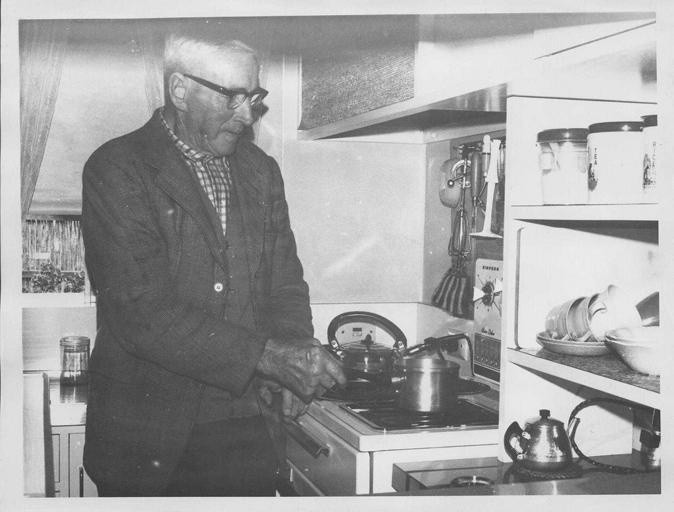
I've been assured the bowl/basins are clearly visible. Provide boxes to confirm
[546,285,643,341]
[606,324,660,376]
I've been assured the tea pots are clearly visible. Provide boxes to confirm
[503,408,582,471]
[323,311,409,393]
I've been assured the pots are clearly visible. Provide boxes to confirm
[389,358,460,415]
[390,332,474,414]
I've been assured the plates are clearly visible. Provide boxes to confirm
[536,329,616,357]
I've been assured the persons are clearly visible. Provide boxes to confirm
[79,26,349,496]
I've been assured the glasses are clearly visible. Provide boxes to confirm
[185,72,270,111]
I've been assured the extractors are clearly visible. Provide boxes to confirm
[296,82,509,149]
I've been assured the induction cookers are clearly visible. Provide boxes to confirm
[301,373,501,432]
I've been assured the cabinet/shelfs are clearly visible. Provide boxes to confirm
[51,426,97,497]
[501,22,673,409]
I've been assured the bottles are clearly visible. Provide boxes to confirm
[539,114,658,206]
[59,336,93,386]
